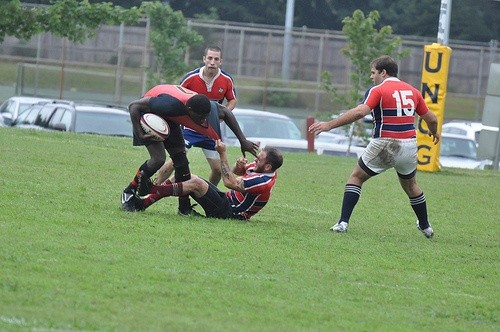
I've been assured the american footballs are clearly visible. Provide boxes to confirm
[141,113,169,141]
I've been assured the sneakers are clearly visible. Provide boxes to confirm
[137,170,152,198]
[414,218,434,239]
[177,207,204,217]
[120,191,132,212]
[124,188,143,212]
[328,221,348,232]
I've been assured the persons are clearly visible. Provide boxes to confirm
[151,45,238,189]
[120,145,283,221]
[308,55,439,238]
[121,83,259,217]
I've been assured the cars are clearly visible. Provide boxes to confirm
[313,113,500,173]
[221,107,314,153]
[0,96,133,137]
